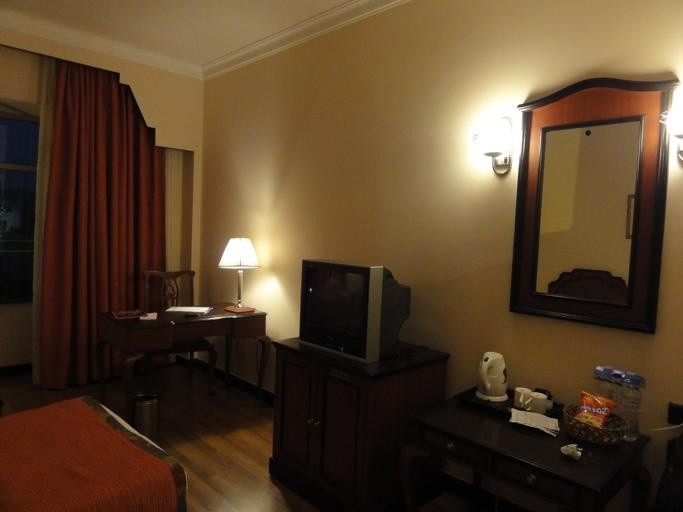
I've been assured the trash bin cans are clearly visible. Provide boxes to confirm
[129,392,160,446]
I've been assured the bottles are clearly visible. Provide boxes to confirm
[592,365,643,444]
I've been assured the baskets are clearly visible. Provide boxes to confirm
[563,403,630,446]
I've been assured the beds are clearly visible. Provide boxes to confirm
[548,269,628,304]
[0,395,187,512]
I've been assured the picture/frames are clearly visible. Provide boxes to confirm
[626,195,635,239]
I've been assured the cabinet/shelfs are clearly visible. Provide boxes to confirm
[269,338,450,512]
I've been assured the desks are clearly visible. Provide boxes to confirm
[406,387,650,512]
[96,303,270,428]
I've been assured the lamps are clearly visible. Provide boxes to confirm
[666,107,683,160]
[218,238,260,314]
[486,131,510,175]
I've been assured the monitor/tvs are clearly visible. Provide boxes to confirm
[297,258,412,362]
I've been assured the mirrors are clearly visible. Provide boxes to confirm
[510,78,680,333]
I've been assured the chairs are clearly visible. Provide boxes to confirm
[142,270,217,397]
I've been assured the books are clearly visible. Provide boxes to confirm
[139,312,157,321]
[165,306,213,315]
[111,308,146,320]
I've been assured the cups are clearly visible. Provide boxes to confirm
[514,386,532,409]
[524,392,547,414]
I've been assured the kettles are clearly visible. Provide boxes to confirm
[475,351,509,402]
